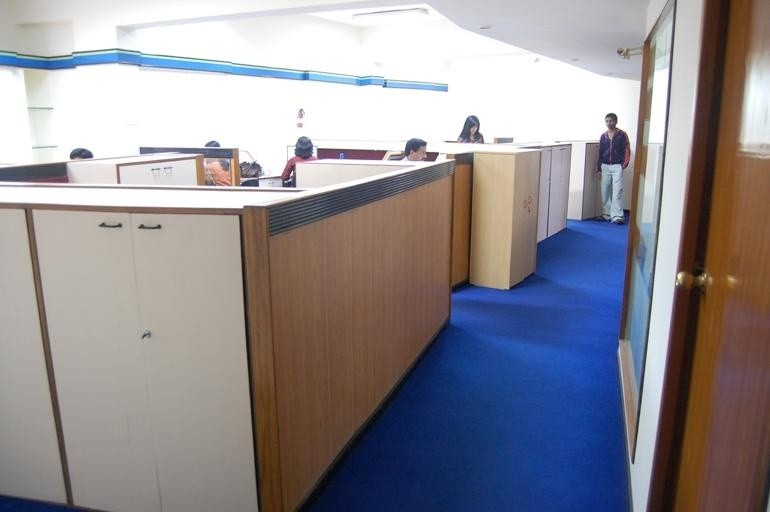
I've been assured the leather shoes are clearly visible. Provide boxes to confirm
[615,218,625,224]
[595,216,605,221]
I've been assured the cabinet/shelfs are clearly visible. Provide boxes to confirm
[466,148,541,289]
[258,175,283,188]
[515,140,604,243]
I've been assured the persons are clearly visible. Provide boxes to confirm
[204,141,231,187]
[69,146,93,160]
[281,135,320,187]
[593,112,631,225]
[457,115,483,143]
[401,137,428,162]
